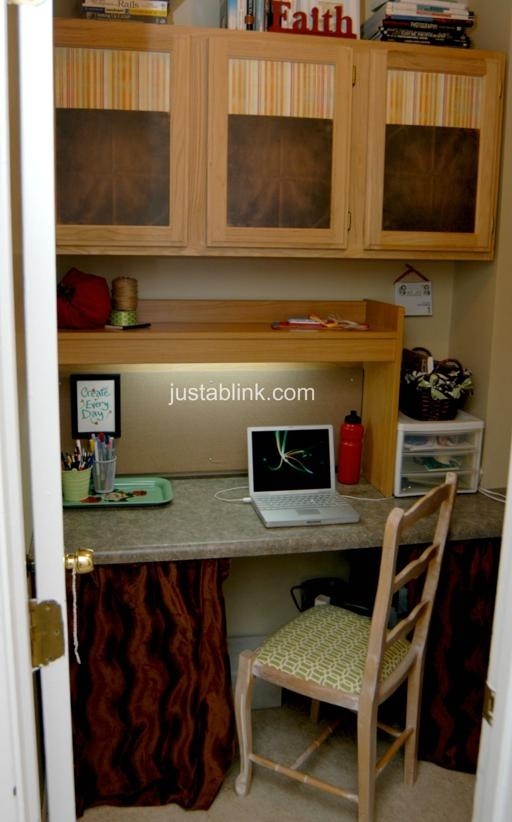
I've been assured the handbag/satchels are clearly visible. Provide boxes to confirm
[57,265,111,328]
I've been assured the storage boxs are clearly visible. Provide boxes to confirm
[394,409,485,500]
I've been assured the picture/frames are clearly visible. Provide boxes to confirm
[69,373,122,440]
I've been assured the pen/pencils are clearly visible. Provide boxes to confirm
[61,433,115,491]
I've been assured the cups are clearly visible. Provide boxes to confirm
[62,466,92,501]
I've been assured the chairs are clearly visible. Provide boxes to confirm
[229,470,463,820]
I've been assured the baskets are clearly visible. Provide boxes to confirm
[400,348,464,420]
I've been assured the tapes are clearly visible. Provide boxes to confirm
[110,310,137,326]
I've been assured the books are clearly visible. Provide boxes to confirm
[220,0,477,48]
[80,0,169,23]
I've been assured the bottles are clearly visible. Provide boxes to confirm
[336,406,365,487]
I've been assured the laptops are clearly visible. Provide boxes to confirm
[245,422,362,528]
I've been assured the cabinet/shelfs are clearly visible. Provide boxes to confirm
[52,16,508,265]
